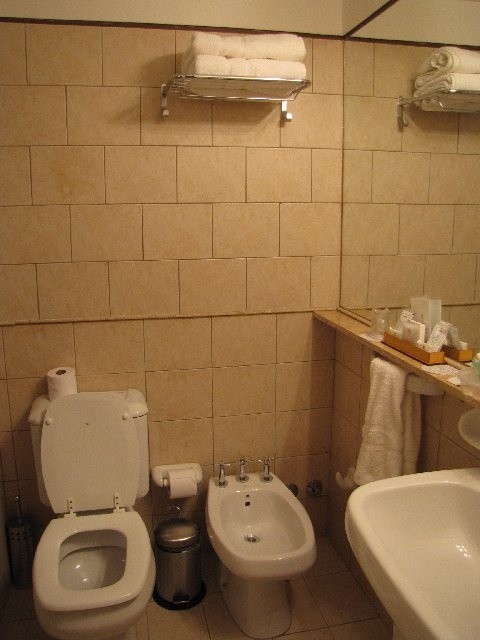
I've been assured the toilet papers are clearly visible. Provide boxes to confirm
[166,467,197,498]
[46,366,78,401]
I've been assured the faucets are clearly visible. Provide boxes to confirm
[236,459,249,483]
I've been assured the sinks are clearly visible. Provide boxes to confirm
[206,471,318,640]
[344,467,480,640]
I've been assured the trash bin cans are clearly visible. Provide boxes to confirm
[153,518,205,611]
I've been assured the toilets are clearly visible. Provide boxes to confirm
[32,393,156,640]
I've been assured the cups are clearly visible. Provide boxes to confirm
[369,307,389,340]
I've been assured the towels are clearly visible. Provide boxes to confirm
[408,46,480,115]
[352,357,423,487]
[181,31,307,104]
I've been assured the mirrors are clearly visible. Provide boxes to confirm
[341,0,480,387]
